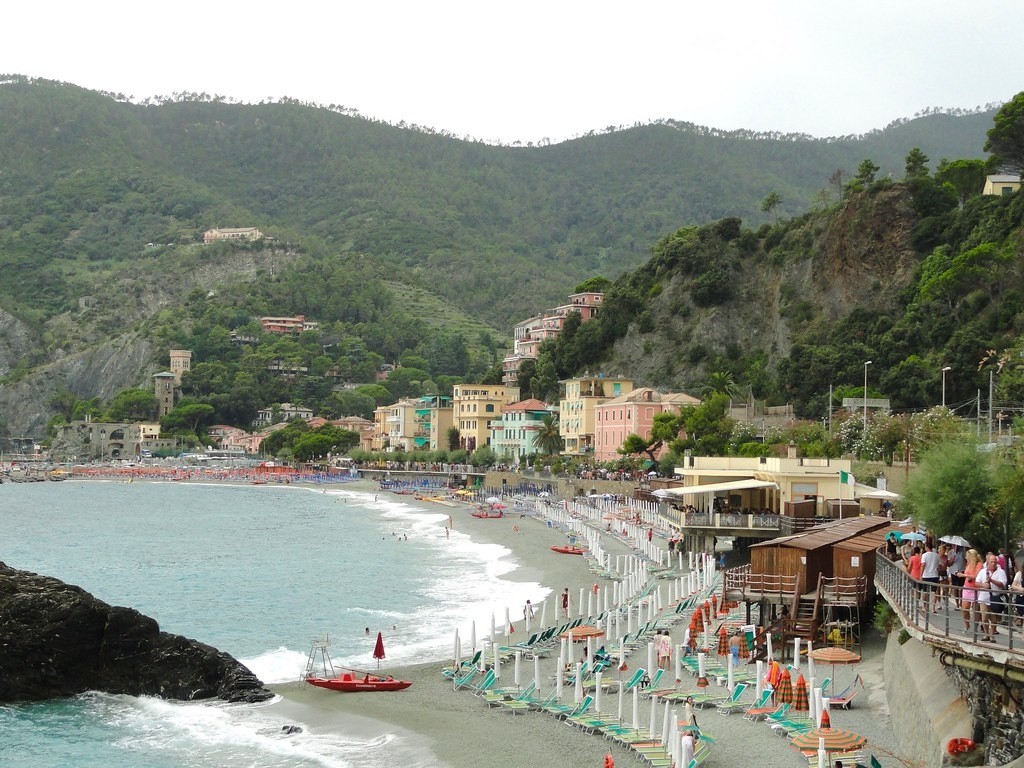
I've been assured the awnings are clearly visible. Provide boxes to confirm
[652,480,780,494]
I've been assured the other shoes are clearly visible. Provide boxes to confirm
[990,636,996,643]
[962,624,999,634]
[981,636,990,641]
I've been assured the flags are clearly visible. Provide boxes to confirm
[841,471,855,485]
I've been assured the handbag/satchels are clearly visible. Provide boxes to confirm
[990,593,1008,613]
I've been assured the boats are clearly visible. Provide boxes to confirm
[551,547,582,555]
[480,515,501,518]
[394,491,413,494]
[473,513,486,516]
[306,671,413,691]
[251,480,268,483]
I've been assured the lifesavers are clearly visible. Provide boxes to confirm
[604,753,613,768]
[593,584,598,593]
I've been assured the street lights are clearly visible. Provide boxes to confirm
[942,367,951,406]
[864,360,872,441]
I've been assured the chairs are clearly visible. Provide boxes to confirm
[443,589,881,768]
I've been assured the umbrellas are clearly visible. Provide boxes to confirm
[938,534,971,548]
[918,534,926,540]
[884,530,902,540]
[900,533,920,540]
[71,458,867,767]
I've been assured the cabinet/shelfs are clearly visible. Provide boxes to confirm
[825,621,862,664]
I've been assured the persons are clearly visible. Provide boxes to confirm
[333,458,1024,727]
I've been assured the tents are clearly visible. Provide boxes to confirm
[865,489,899,500]
